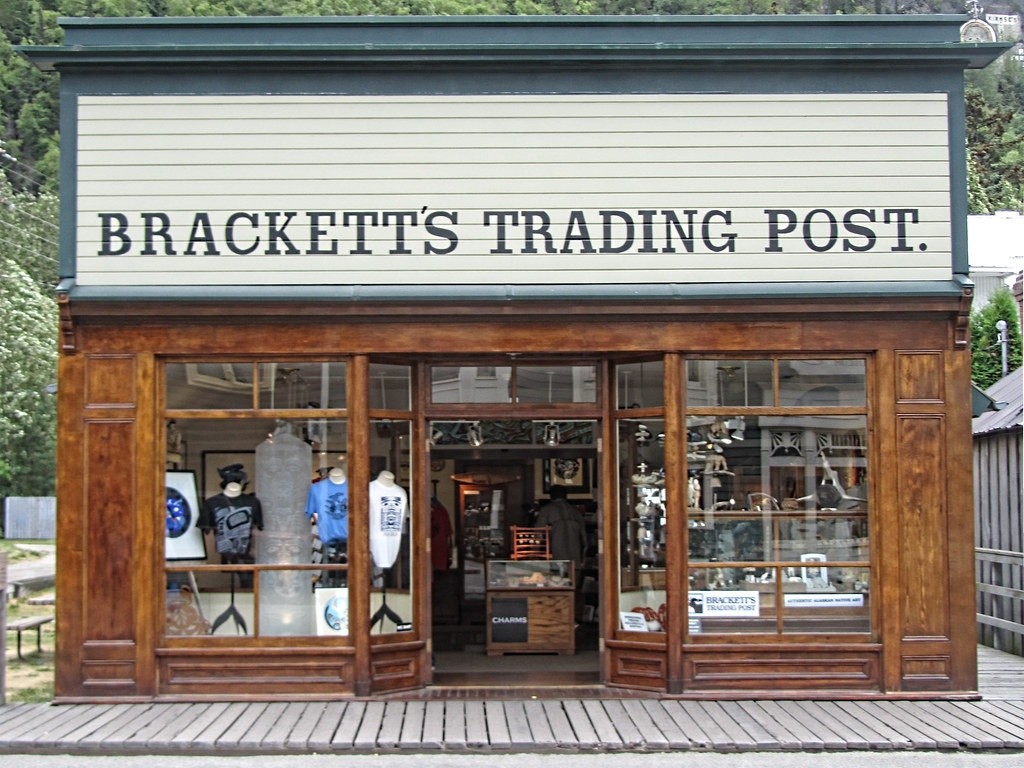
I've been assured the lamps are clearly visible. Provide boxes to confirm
[544,423,561,448]
[468,425,483,446]
[432,430,443,447]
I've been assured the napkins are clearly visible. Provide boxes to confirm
[720,419,747,445]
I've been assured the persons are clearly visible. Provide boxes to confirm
[196,480,263,555]
[534,483,588,632]
[732,483,860,584]
[369,469,410,569]
[305,466,349,546]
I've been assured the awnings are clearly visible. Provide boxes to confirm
[972,380,1009,418]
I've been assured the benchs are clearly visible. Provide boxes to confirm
[4,615,53,660]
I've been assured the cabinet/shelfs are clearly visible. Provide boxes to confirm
[485,557,578,656]
